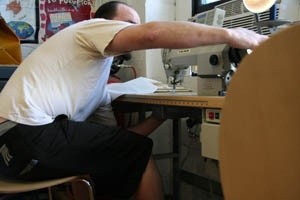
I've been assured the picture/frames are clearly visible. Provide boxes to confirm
[38,1,97,47]
[0,0,40,44]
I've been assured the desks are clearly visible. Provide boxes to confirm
[110,89,222,200]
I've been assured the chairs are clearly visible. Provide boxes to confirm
[0,176,96,200]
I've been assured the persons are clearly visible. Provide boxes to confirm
[0,1,271,200]
